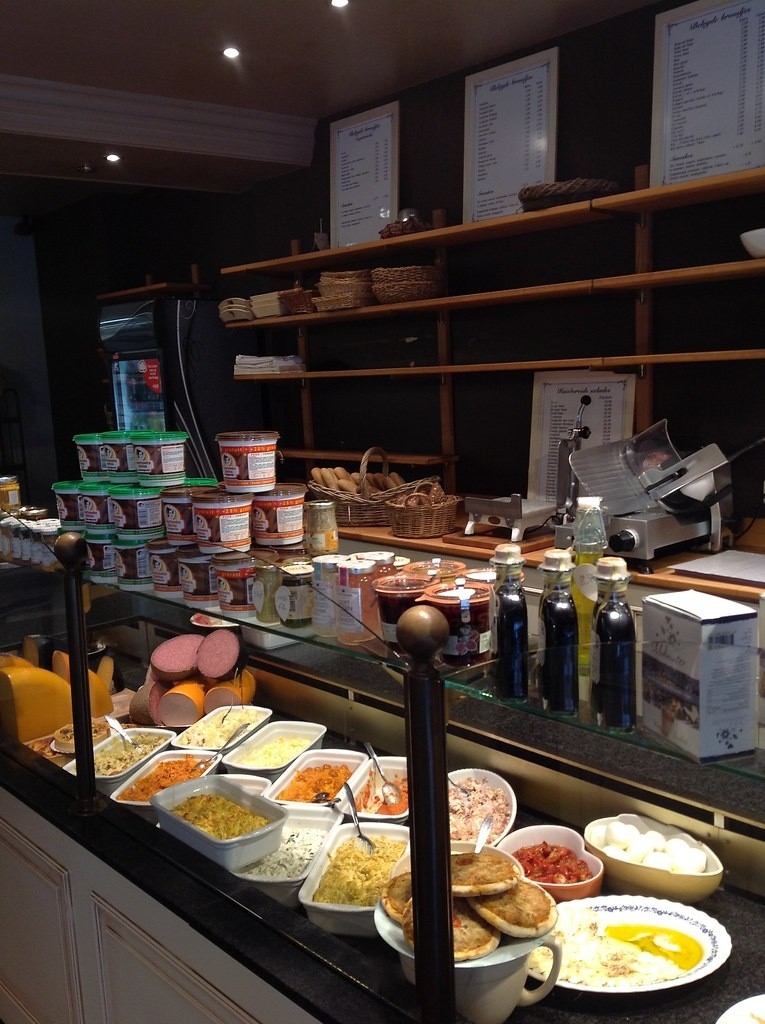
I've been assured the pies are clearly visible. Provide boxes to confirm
[379,852,559,966]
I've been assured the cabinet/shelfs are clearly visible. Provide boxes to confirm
[217,167,764,489]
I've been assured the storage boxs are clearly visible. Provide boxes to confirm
[643,587,759,766]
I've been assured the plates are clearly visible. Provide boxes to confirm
[527,895,734,994]
[715,989,765,1024]
[189,612,239,629]
[218,291,285,322]
[373,896,551,969]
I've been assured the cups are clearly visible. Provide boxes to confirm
[372,558,525,671]
[128,668,256,724]
[49,428,307,620]
[400,935,562,1024]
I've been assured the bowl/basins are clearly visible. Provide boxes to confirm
[739,228,765,258]
[61,702,725,939]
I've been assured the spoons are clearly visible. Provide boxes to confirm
[190,723,250,772]
[364,741,402,805]
[343,781,375,854]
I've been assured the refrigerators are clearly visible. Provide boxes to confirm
[94,280,265,481]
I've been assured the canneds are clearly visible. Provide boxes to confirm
[0,474,64,574]
[252,499,411,644]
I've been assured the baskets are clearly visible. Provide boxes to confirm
[310,271,376,311]
[385,482,464,539]
[371,264,444,315]
[379,218,433,238]
[279,288,318,314]
[307,446,398,526]
[519,180,618,211]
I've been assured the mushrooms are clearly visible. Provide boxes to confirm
[589,819,708,876]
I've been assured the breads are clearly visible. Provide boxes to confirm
[309,466,454,505]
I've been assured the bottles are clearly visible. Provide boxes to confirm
[0,475,61,573]
[255,498,410,648]
[486,543,529,704]
[588,557,637,737]
[566,496,609,677]
[535,548,580,719]
